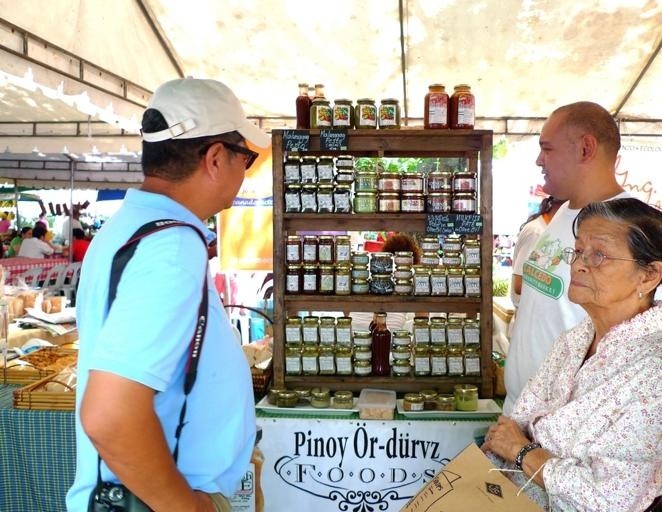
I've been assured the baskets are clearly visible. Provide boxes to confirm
[224,304,273,396]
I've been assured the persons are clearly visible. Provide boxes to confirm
[509,196,568,343]
[499,101,640,417]
[479,197,662,512]
[0,208,92,262]
[64,78,273,512]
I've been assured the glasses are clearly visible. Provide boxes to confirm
[198,140,259,169]
[559,246,638,267]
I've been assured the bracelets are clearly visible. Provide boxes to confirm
[515,441,542,471]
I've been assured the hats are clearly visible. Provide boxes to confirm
[140,76,271,148]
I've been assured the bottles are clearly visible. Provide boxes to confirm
[369,311,392,378]
[295,83,325,128]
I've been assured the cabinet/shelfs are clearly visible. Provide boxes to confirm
[271,129,492,397]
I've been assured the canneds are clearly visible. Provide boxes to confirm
[310,97,400,129]
[423,84,449,129]
[285,315,372,376]
[449,84,475,128]
[286,235,480,296]
[403,383,478,412]
[267,386,354,409]
[391,316,481,377]
[283,155,477,213]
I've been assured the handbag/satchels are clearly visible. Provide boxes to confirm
[399,443,548,512]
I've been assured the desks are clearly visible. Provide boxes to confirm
[0,254,513,512]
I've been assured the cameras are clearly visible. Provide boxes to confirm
[89,483,153,512]
